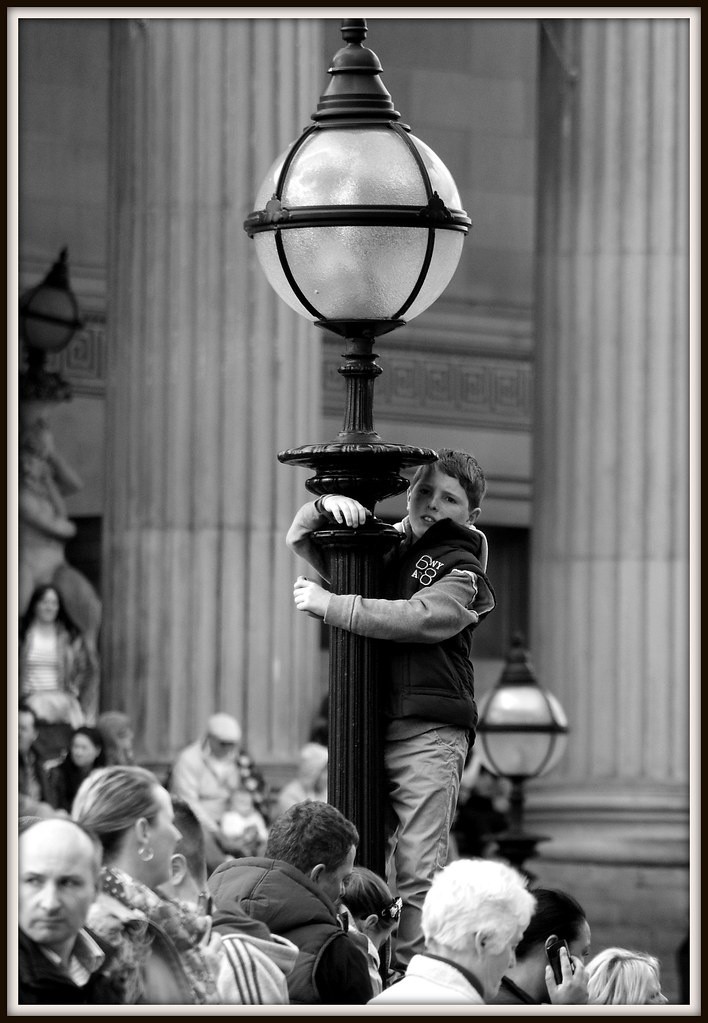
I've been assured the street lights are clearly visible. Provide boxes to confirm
[239,17,473,879]
[477,646,568,861]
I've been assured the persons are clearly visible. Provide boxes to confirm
[71,765,223,1004]
[207,801,374,1005]
[335,865,401,997]
[366,858,538,1005]
[158,795,300,1005]
[485,888,591,1005]
[18,424,102,638]
[18,703,135,814]
[18,586,87,711]
[18,818,128,1005]
[584,946,669,1005]
[285,448,498,972]
[165,712,328,858]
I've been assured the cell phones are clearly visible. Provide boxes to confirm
[546,940,575,986]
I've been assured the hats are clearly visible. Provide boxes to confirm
[209,714,239,742]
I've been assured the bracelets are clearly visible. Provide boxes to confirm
[322,494,337,506]
